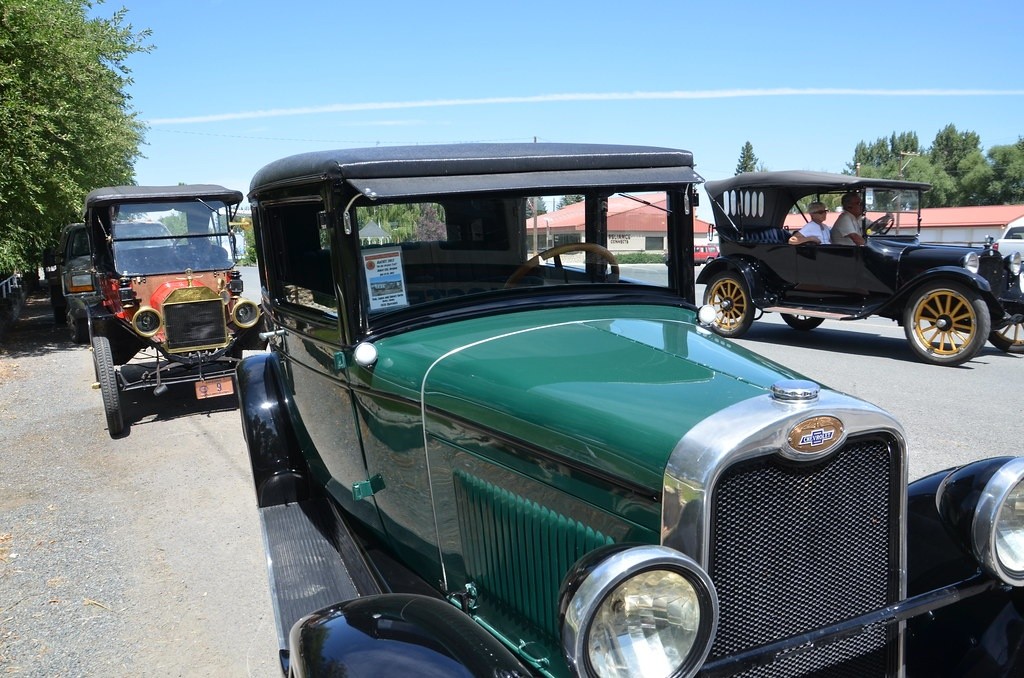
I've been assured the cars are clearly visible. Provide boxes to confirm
[46,183,270,438]
[993,215,1024,266]
[697,168,1024,360]
[232,139,1024,678]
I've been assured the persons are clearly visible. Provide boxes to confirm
[830,192,893,246]
[787,202,834,245]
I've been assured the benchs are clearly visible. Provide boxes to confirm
[726,226,794,245]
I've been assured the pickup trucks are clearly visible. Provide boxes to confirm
[666,246,719,266]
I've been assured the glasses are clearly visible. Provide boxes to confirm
[812,209,827,214]
[857,202,864,206]
